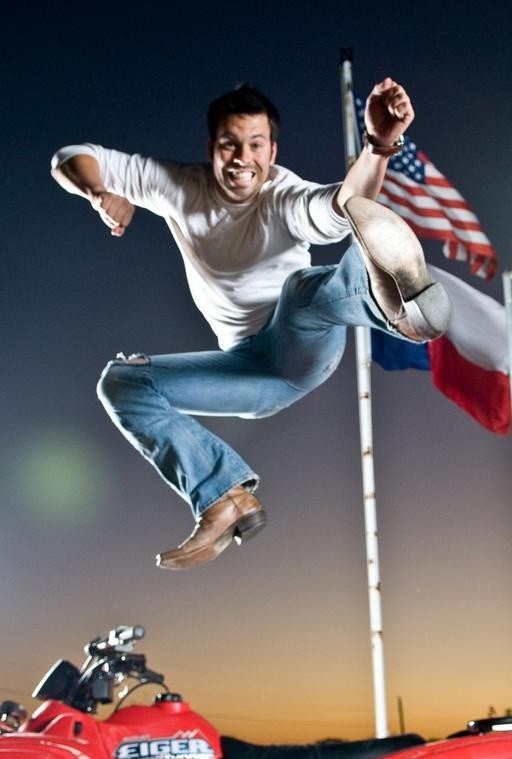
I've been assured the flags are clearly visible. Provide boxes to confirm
[371,262,512,435]
[353,91,495,282]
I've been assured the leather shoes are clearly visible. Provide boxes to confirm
[155,485,268,571]
[342,193,453,344]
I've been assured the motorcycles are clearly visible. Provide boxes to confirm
[0,624,512,759]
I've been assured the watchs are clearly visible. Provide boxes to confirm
[361,129,405,157]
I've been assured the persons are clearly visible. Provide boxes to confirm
[50,77,454,572]
